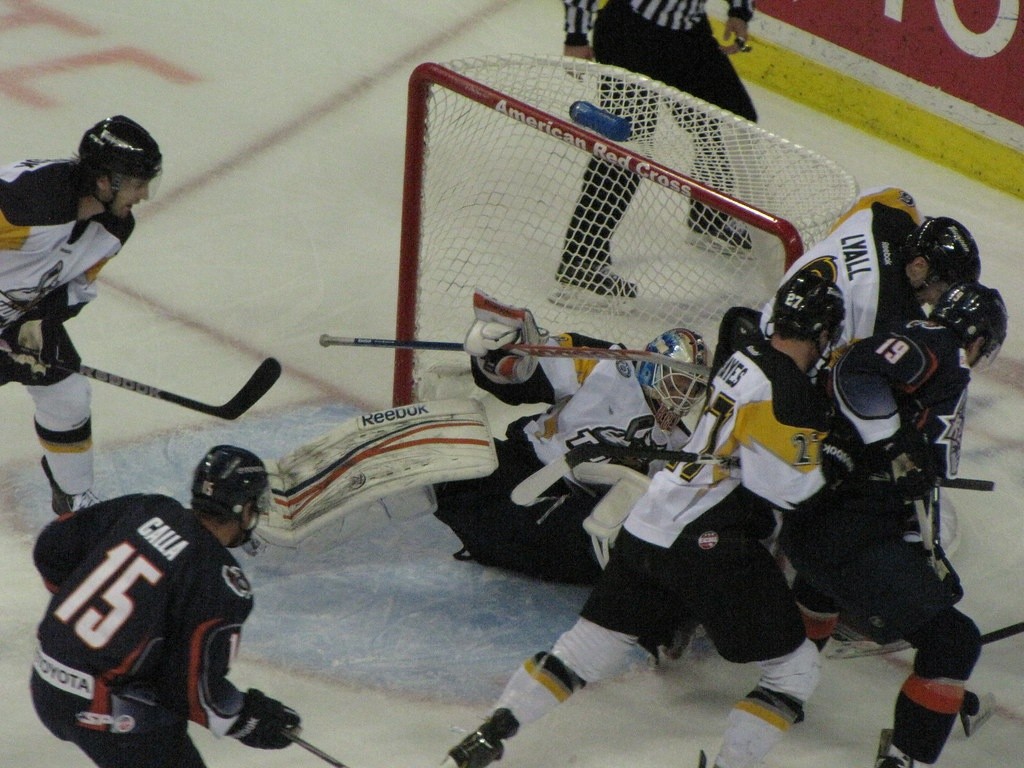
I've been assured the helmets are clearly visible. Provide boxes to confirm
[190,445,270,512]
[771,275,847,349]
[903,217,981,305]
[931,280,1009,373]
[78,115,162,180]
[638,328,710,430]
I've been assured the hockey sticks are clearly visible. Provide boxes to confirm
[319,333,712,387]
[978,618,1022,645]
[509,441,996,508]
[280,730,347,767]
[1,341,283,421]
[912,497,1000,737]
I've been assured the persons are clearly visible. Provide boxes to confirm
[256,188,1008,768]
[30,445,303,768]
[548,1,758,310]
[0,115,163,513]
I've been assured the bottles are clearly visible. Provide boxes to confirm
[569,100,632,140]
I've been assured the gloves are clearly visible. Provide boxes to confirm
[0,315,60,384]
[225,688,301,750]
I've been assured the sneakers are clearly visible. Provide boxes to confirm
[439,708,519,768]
[548,247,640,314]
[40,454,101,519]
[639,629,690,673]
[683,195,753,259]
